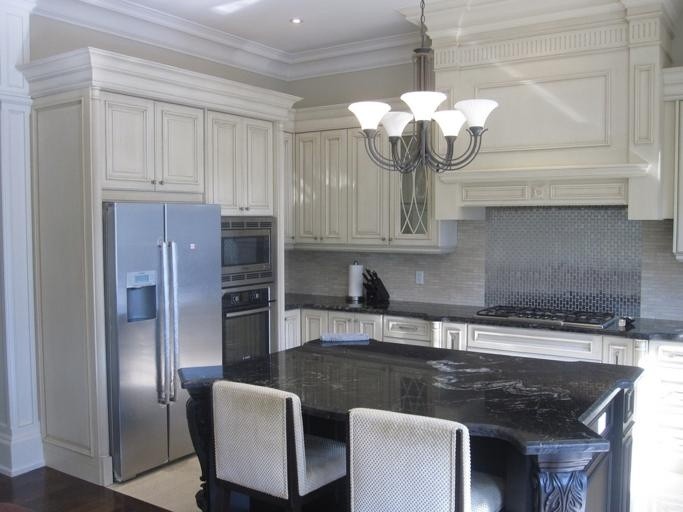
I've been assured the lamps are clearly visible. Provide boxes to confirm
[348,0,499,173]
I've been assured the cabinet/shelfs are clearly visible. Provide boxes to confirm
[349,124,457,254]
[283,304,301,351]
[301,306,328,344]
[293,128,348,251]
[104,99,204,195]
[204,108,273,217]
[328,309,383,342]
[637,332,682,512]
[385,315,435,348]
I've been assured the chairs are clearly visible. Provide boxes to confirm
[348,407,508,512]
[209,379,346,512]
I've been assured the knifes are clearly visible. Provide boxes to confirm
[360,268,377,290]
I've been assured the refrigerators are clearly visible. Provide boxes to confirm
[101,201,228,485]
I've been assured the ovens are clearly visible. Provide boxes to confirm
[222,291,272,362]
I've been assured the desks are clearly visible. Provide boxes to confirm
[178,339,644,512]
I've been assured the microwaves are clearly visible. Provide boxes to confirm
[220,215,277,288]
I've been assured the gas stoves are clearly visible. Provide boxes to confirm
[474,300,617,329]
